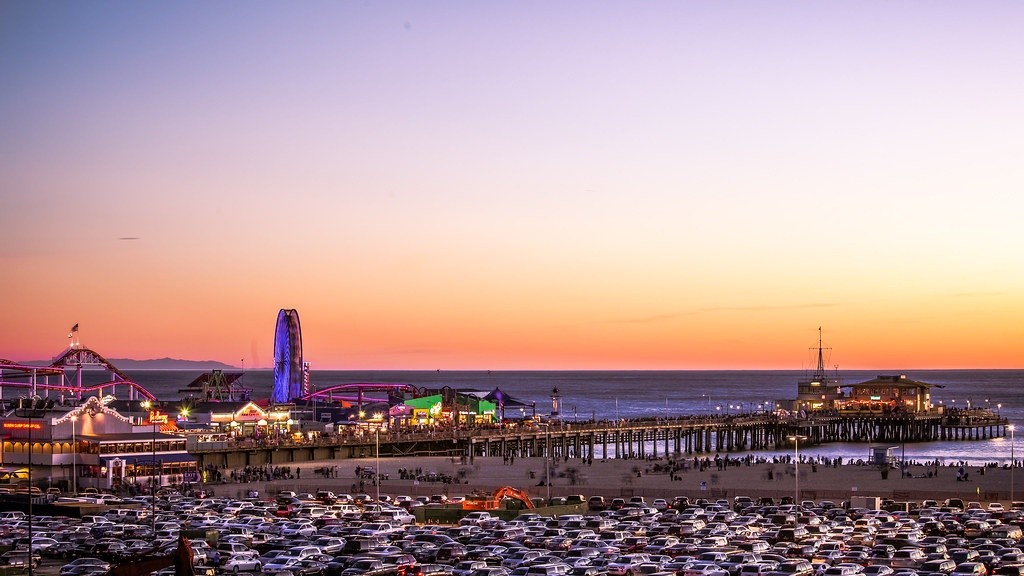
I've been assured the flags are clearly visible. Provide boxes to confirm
[71,323,78,332]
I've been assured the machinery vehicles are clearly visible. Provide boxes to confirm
[462,487,535,510]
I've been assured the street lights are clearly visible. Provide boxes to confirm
[182,407,191,474]
[70,416,77,494]
[9,396,55,576]
[787,435,808,527]
[702,394,710,417]
[141,401,156,532]
[1008,424,1015,502]
[370,427,387,511]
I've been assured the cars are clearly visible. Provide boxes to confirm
[0,484,1024,576]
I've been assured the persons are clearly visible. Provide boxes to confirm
[208,405,1024,492]
[214,552,220,569]
